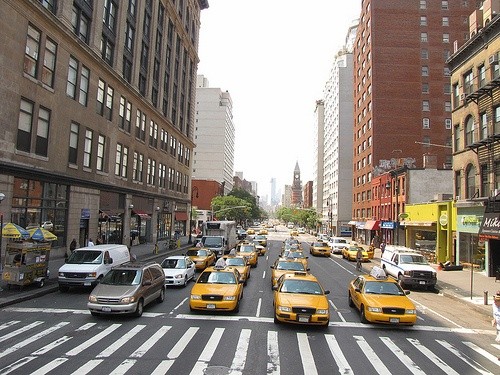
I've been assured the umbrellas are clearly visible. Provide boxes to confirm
[0,223,30,241]
[27,227,57,241]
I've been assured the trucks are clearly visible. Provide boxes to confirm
[58,243,131,291]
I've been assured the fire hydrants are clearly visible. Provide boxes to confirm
[484,290,489,305]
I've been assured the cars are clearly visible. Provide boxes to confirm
[416,233,425,239]
[476,246,485,259]
[188,258,244,313]
[160,256,197,287]
[236,219,305,240]
[348,266,417,327]
[181,235,311,291]
[310,238,331,257]
[87,260,166,317]
[26,221,64,230]
[316,234,374,262]
[273,271,330,328]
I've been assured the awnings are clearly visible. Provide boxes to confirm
[176,211,187,220]
[478,212,500,240]
[348,221,366,229]
[363,221,379,230]
[133,210,151,218]
[100,209,121,219]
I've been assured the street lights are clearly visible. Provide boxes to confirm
[327,197,333,237]
[188,186,199,245]
[386,170,400,246]
[212,206,246,221]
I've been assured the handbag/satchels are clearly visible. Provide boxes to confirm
[70,245,76,250]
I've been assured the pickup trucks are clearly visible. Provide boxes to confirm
[381,245,437,289]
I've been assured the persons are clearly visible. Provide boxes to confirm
[88,238,94,247]
[357,248,362,260]
[13,253,26,265]
[380,240,385,257]
[70,238,76,252]
[371,238,375,246]
